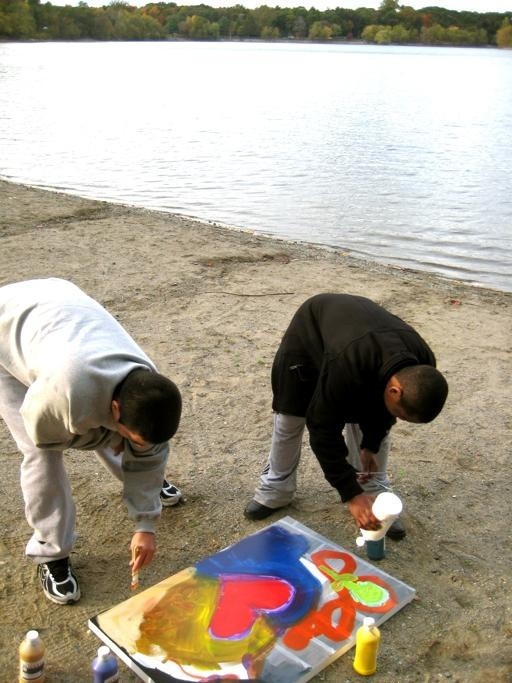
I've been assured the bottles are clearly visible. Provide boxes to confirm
[355,492,402,547]
[92,644,119,682]
[17,630,44,683]
[354,616,380,676]
[365,536,387,560]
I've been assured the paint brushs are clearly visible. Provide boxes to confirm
[356,471,388,476]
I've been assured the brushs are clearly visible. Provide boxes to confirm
[130,545,140,590]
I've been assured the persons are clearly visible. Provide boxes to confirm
[231,293,451,541]
[1,277,186,607]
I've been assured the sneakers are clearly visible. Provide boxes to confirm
[242,499,278,518]
[388,520,405,540]
[158,480,183,507]
[38,557,82,607]
